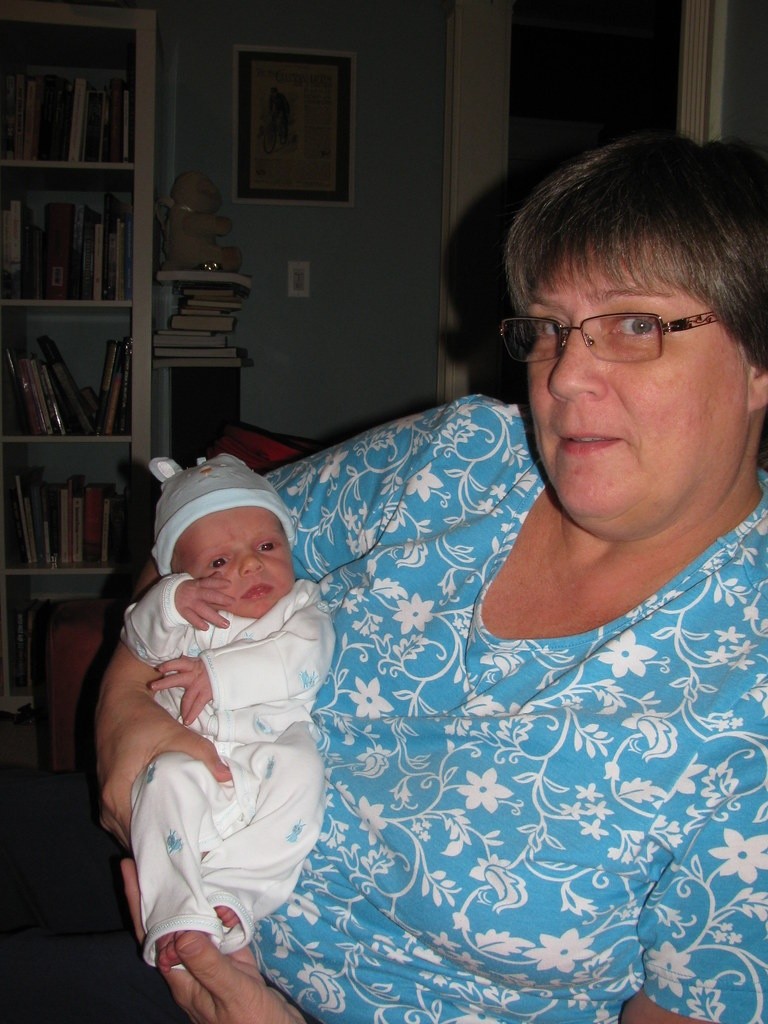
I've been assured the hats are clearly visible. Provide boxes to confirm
[146,454,295,574]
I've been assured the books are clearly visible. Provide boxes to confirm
[153,263,255,371]
[1,58,131,695]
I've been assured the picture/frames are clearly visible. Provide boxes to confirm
[234,45,356,207]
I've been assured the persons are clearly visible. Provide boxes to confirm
[90,118,767,1023]
[117,443,337,964]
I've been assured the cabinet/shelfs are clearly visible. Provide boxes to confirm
[0,0,156,713]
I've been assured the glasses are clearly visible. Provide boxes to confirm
[497,302,719,365]
